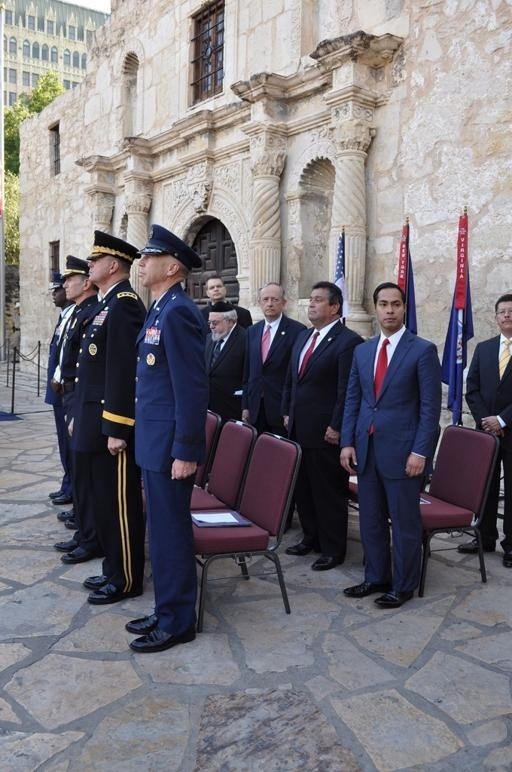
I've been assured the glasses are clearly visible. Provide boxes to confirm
[207,317,229,326]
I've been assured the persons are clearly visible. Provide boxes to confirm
[458,294,512,568]
[57,254,100,528]
[44,273,75,505]
[285,281,364,570]
[125,224,206,653]
[338,282,441,609]
[198,275,254,334]
[240,282,309,531]
[84,230,144,606]
[205,303,247,419]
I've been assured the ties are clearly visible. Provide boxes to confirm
[369,339,390,437]
[498,340,512,381]
[210,338,224,369]
[261,325,272,364]
[298,332,320,381]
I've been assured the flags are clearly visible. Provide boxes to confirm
[398,223,417,336]
[440,215,476,426]
[334,232,350,326]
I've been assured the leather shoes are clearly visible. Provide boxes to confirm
[458,538,496,554]
[48,489,105,565]
[82,574,128,604]
[125,612,197,653]
[287,543,323,556]
[374,589,413,607]
[342,580,392,598]
[311,554,345,570]
[504,551,511,567]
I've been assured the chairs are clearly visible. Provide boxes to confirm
[419,425,500,599]
[345,424,441,565]
[192,431,302,634]
[196,410,223,488]
[141,420,258,582]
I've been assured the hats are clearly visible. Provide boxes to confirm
[47,223,203,291]
[208,300,235,312]
